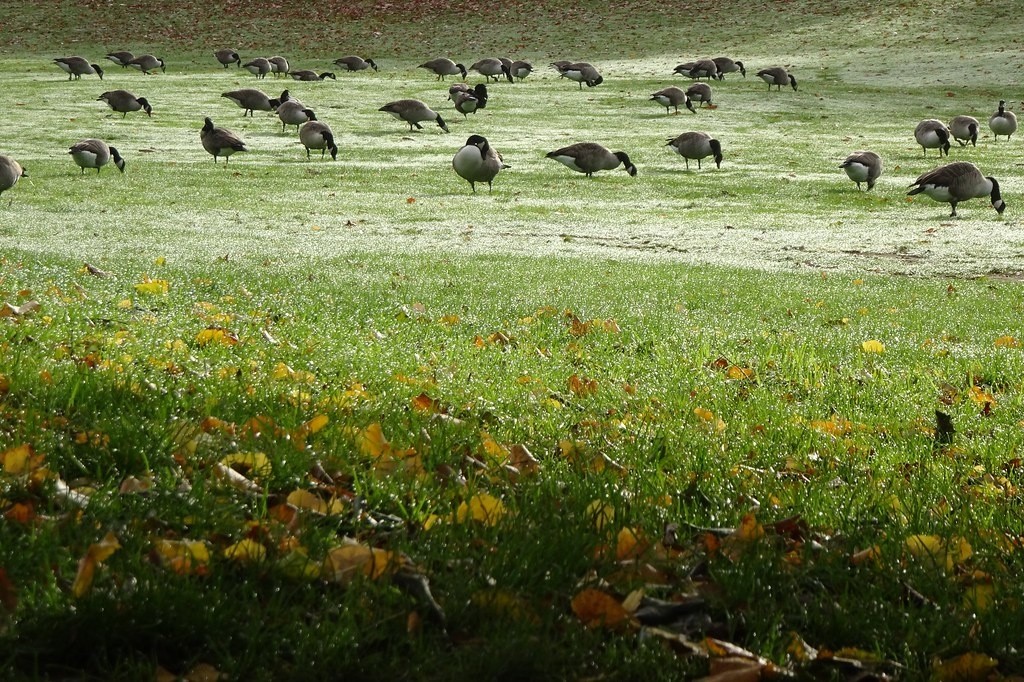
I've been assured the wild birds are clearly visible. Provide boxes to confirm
[200,117,246,164]
[213,49,241,68]
[647,82,712,116]
[550,60,604,90]
[0,155,28,195]
[452,135,512,193]
[97,90,152,120]
[836,97,1024,222]
[469,57,534,84]
[378,99,450,133]
[68,139,126,174]
[241,56,338,81]
[672,57,797,92]
[447,83,488,120]
[664,132,723,170]
[332,55,378,73]
[545,143,637,178]
[416,57,467,82]
[220,89,338,161]
[52,49,167,82]
